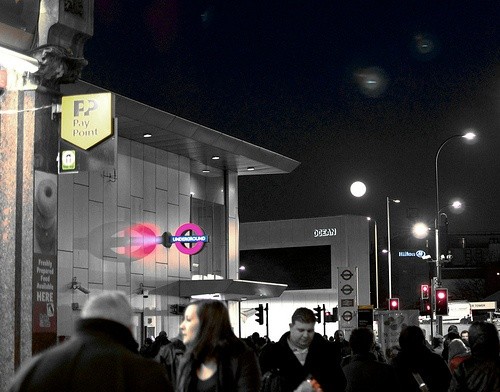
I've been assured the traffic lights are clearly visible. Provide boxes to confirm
[312,305,321,323]
[421,285,429,299]
[255,304,263,324]
[389,299,400,310]
[435,288,449,316]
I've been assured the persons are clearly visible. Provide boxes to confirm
[139,300,500,392]
[5,289,173,392]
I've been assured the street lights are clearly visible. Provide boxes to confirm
[386,197,401,298]
[435,132,476,335]
[366,216,379,308]
